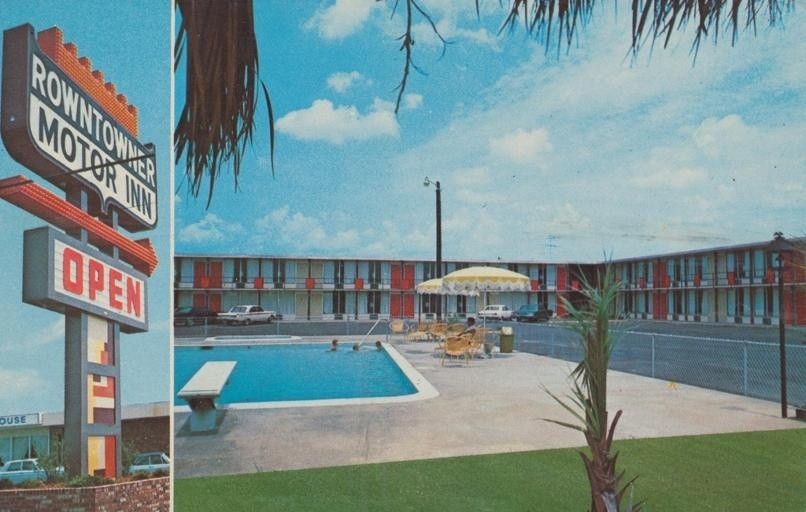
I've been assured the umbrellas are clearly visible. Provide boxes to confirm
[414,278,481,316]
[443,265,531,335]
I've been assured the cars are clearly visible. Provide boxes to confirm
[216,305,276,325]
[175,307,217,327]
[479,304,513,320]
[512,303,548,321]
[128,451,170,474]
[0,459,66,486]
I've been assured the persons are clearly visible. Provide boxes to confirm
[330,339,340,351]
[440,316,477,359]
[374,341,382,349]
[353,344,362,352]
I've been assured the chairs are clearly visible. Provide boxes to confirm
[386,317,492,368]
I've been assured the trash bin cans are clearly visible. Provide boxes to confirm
[500,325,515,353]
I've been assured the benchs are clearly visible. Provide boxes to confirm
[177,360,241,433]
